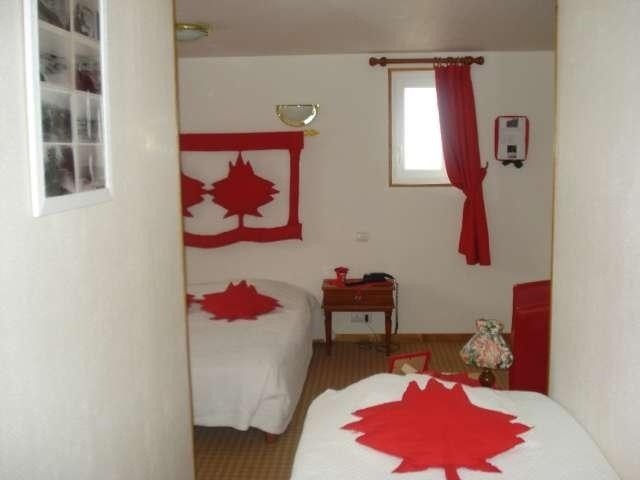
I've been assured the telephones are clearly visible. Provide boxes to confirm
[364,273,385,281]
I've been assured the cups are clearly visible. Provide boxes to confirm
[334,267,348,287]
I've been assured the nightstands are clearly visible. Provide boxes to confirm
[320,279,395,356]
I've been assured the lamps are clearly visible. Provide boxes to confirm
[276,104,316,126]
[175,23,208,42]
[459,318,513,390]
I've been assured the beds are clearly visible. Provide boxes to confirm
[185,278,317,443]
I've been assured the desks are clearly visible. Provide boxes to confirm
[292,372,620,480]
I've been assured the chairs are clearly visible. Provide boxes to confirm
[509,279,550,395]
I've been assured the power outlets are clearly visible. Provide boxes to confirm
[349,311,372,322]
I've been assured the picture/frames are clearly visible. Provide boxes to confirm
[23,0,115,221]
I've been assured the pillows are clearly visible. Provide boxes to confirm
[188,279,311,308]
